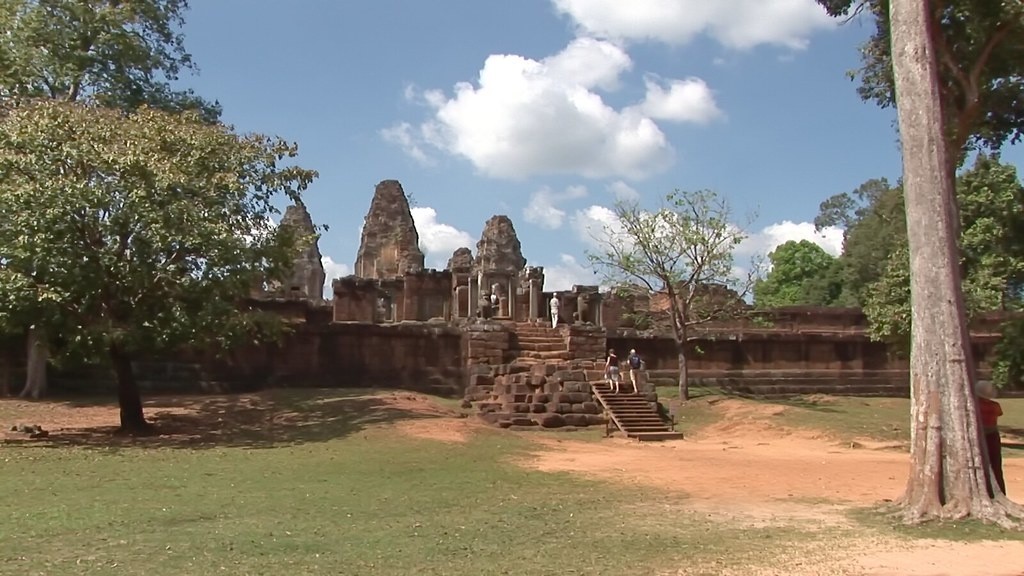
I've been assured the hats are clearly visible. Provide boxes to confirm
[629,349,636,354]
[553,293,557,295]
[608,348,616,353]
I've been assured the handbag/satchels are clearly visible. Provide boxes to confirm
[604,373,608,380]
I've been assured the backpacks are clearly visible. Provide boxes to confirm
[630,355,640,369]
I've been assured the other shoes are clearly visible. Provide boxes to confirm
[632,392,639,395]
[610,389,614,391]
[615,391,619,394]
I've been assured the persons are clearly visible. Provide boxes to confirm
[628,348,642,395]
[549,293,560,329]
[605,349,622,395]
[975,380,1006,496]
